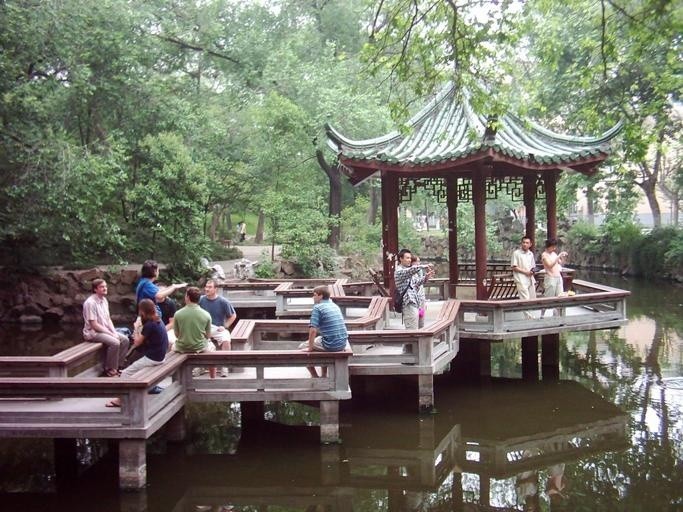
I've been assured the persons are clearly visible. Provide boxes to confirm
[297,284,348,378]
[510,237,538,321]
[103,297,168,409]
[134,260,188,394]
[392,249,437,365]
[239,221,246,242]
[80,278,130,379]
[192,277,238,377]
[172,285,218,381]
[412,255,431,329]
[236,221,241,241]
[540,238,568,318]
[540,441,569,509]
[513,446,542,511]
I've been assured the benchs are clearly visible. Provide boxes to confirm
[484,268,575,312]
[367,267,394,312]
[450,262,542,288]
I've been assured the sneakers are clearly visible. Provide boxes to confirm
[220,368,229,378]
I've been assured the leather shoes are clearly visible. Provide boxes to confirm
[147,385,165,394]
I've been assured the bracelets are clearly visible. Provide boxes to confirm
[530,267,535,274]
[221,323,229,330]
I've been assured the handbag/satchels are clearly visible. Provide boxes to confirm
[392,291,405,313]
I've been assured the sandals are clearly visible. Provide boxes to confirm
[102,365,121,378]
[103,399,122,410]
